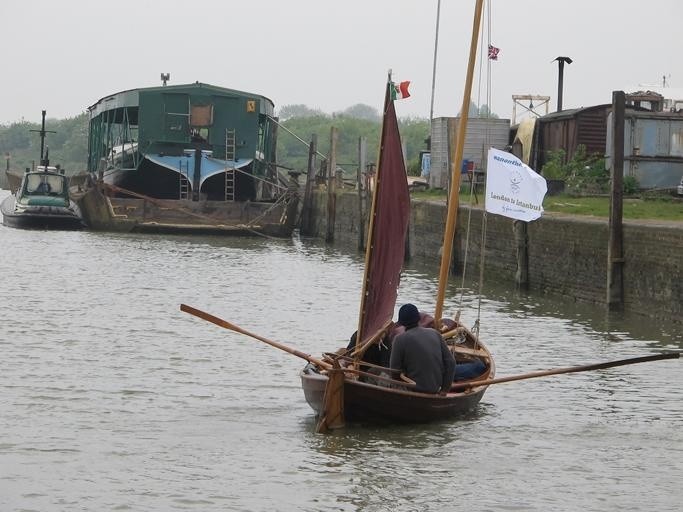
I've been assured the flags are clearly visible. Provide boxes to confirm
[484,147,548,223]
[389,81,410,100]
[488,46,500,61]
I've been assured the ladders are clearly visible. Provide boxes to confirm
[180,160,188,201]
[225,128,235,202]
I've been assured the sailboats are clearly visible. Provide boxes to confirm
[300,0,496,426]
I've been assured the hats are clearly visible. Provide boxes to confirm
[395,304,421,328]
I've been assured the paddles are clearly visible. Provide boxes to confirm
[179,303,333,371]
[449,351,679,390]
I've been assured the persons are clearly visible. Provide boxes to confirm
[347,330,391,382]
[389,303,456,397]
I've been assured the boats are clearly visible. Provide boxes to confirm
[0,146,88,229]
[68,73,301,234]
